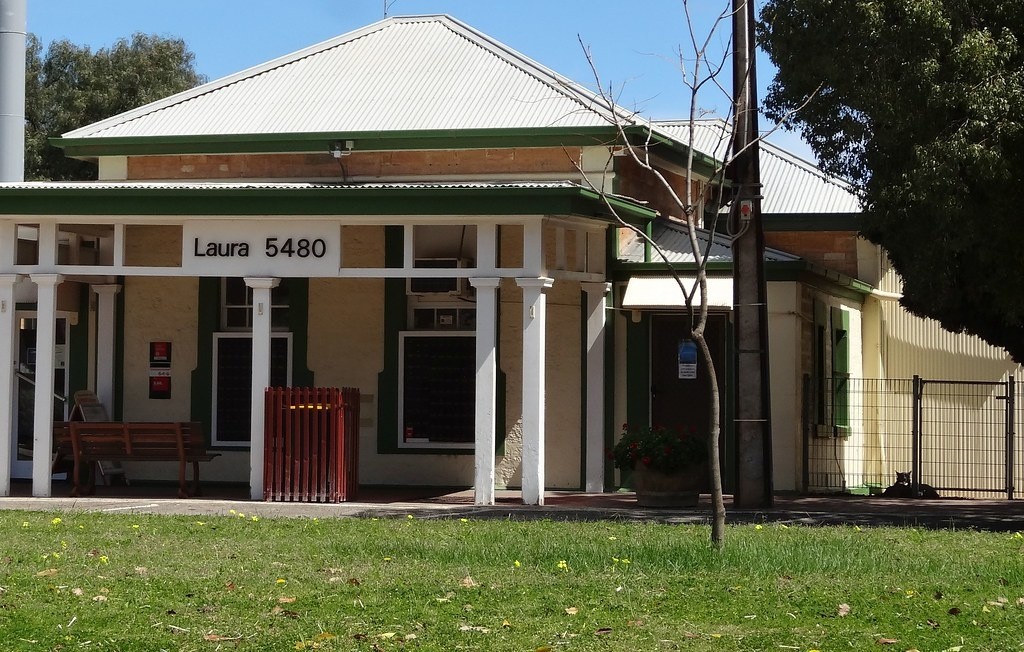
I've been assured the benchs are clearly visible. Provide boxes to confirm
[52,419,223,499]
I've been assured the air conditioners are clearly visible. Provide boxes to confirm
[406,257,475,298]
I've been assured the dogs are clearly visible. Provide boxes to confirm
[885,471,941,499]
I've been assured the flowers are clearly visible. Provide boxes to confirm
[604,421,708,483]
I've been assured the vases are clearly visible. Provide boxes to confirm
[633,458,703,508]
